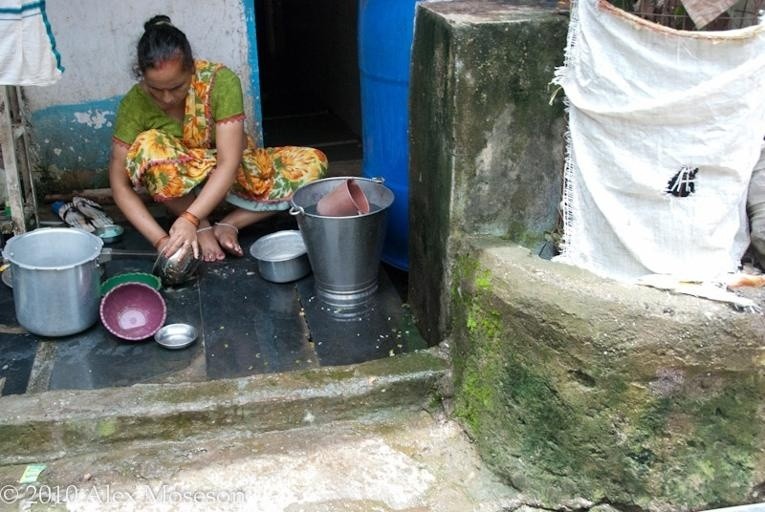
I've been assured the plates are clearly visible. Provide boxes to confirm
[154,322,199,351]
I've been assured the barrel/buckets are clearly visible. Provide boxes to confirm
[288,176,395,306]
[357,1,421,271]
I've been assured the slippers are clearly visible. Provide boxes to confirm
[53,201,96,233]
[72,197,113,229]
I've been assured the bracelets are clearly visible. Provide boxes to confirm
[180,210,200,227]
[154,235,170,251]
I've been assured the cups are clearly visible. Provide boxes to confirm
[315,178,370,218]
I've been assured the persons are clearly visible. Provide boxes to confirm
[107,14,329,263]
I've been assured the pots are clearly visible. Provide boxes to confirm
[249,229,312,285]
[0,227,104,338]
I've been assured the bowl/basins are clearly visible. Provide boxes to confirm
[152,239,203,287]
[93,224,125,246]
[99,281,168,342]
[100,271,162,298]
